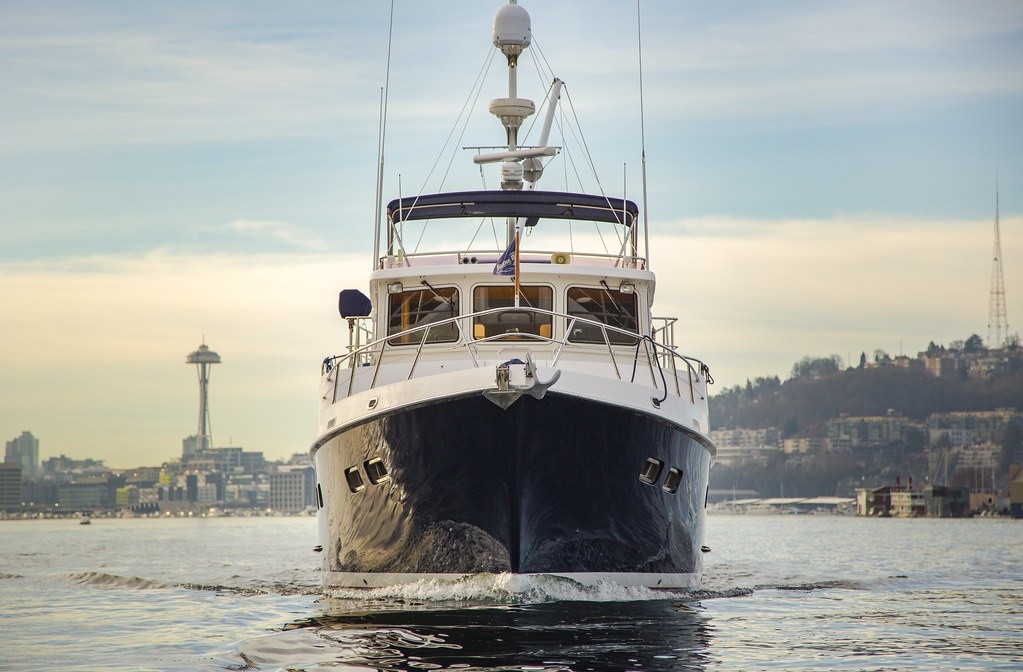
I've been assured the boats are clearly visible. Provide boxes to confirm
[79,515,90,526]
[306,2,716,619]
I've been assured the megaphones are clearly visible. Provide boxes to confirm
[551,253,570,264]
